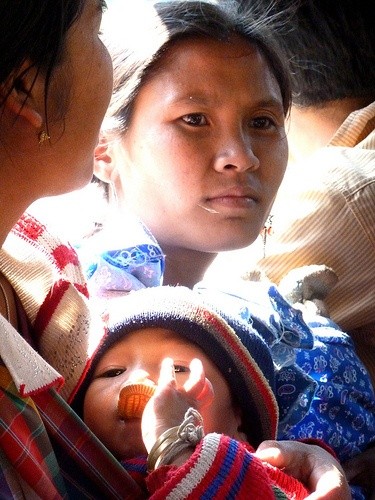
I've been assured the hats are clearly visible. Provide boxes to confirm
[84,285,283,452]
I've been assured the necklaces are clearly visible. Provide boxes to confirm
[0,286,12,323]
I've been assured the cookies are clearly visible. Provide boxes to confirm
[117,384,154,418]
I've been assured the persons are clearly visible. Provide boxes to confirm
[72,1,375,500]
[1,0,350,500]
[231,2,375,368]
[69,284,305,500]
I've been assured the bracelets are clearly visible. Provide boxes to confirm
[145,424,184,469]
